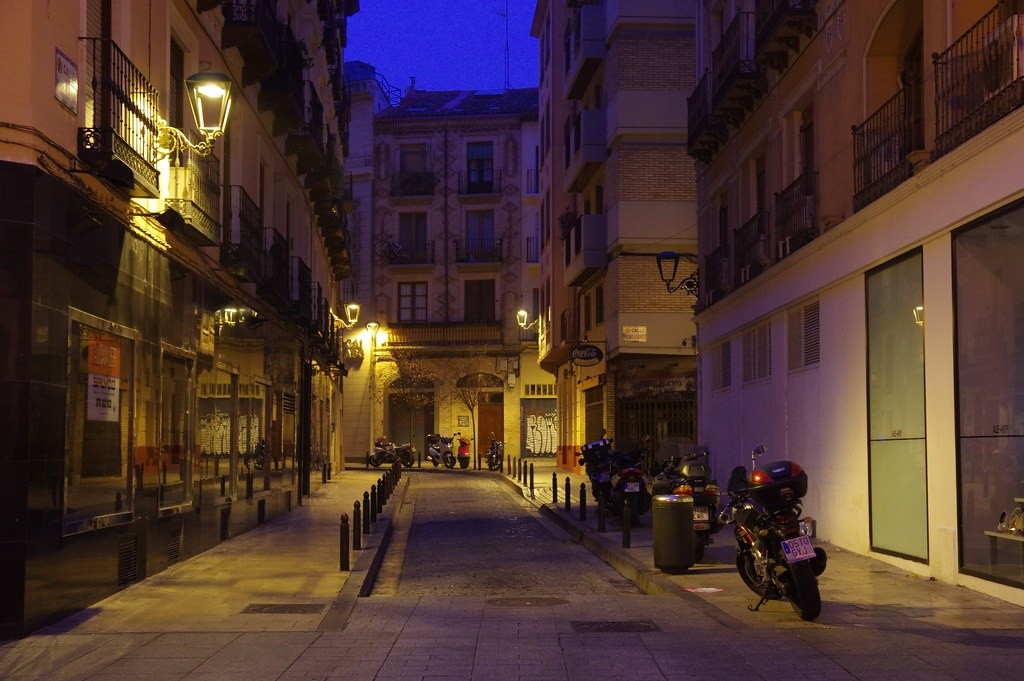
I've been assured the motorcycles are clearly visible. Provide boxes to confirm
[484,432,506,471]
[719,443,826,620]
[661,445,730,563]
[369,436,417,468]
[574,429,652,527]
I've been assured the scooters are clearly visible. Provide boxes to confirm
[426,432,461,469]
[454,434,473,469]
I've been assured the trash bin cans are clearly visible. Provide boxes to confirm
[651,494,694,569]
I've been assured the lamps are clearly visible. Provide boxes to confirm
[656,251,701,300]
[515,308,539,330]
[365,320,381,340]
[333,302,363,330]
[138,72,239,165]
